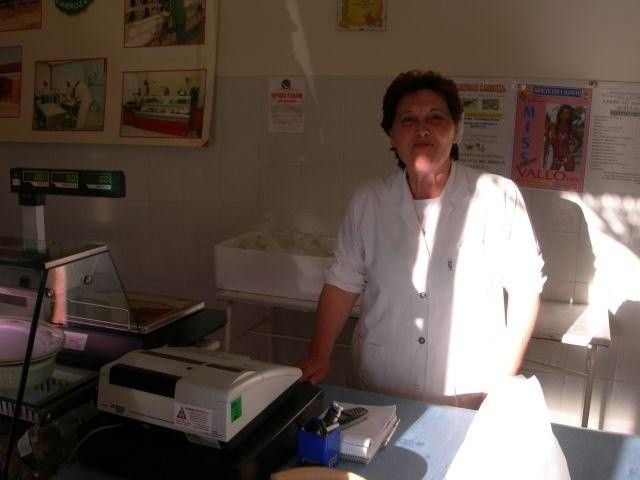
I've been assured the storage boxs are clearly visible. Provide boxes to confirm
[214,231,364,308]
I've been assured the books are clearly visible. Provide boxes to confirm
[319,400,398,465]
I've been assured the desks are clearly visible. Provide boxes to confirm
[216,291,610,428]
[36,104,73,130]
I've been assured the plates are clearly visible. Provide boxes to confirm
[0,314,67,366]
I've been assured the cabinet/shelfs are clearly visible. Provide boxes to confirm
[134,96,191,123]
[0,234,147,479]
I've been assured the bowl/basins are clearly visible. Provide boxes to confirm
[0,354,57,392]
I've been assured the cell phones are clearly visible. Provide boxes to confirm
[338,407,370,429]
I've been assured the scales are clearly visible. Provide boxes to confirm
[0,167,206,362]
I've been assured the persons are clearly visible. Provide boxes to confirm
[543,104,582,170]
[160,1,188,46]
[288,69,548,412]
